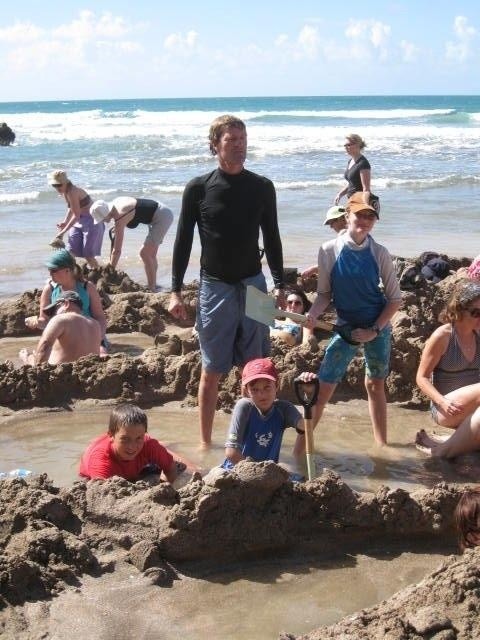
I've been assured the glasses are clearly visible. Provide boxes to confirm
[288,300,301,306]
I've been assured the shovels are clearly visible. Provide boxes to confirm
[282,268,316,285]
[245,285,361,345]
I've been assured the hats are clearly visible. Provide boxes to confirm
[42,170,113,317]
[323,191,380,225]
[242,359,277,386]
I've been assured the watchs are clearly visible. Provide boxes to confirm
[371,324,382,335]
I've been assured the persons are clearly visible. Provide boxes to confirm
[415,281,480,427]
[89,196,173,290]
[416,404,480,460]
[48,171,105,267]
[169,115,285,449]
[302,192,402,445]
[222,359,317,474]
[454,493,480,547]
[40,250,108,351]
[300,205,349,280]
[18,290,101,366]
[270,289,315,344]
[80,405,177,482]
[334,134,371,204]
